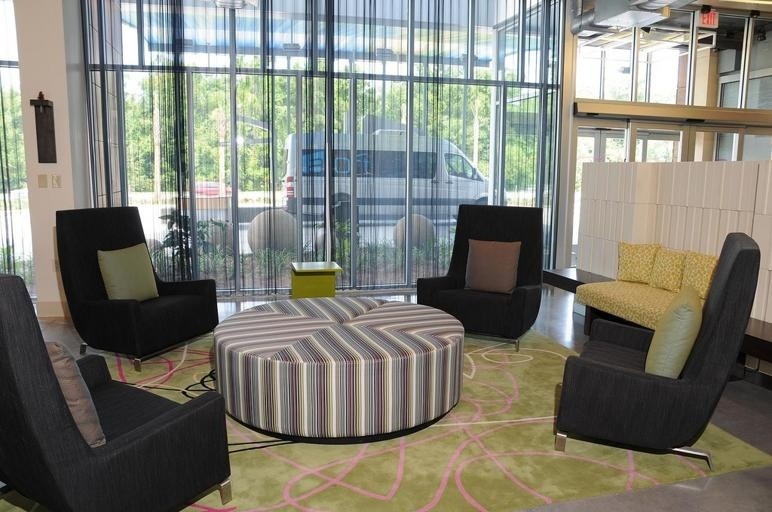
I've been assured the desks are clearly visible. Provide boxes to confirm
[211,294,466,442]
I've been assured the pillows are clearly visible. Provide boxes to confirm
[644,280,704,383]
[42,339,110,449]
[465,235,525,296]
[616,242,658,283]
[679,249,717,301]
[97,242,162,304]
[652,246,685,297]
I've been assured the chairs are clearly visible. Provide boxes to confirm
[54,205,221,373]
[416,204,545,353]
[1,274,234,512]
[554,231,763,473]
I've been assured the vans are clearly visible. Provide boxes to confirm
[282,109,508,228]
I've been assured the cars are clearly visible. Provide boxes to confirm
[193,180,232,196]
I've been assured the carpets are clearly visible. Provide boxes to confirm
[91,313,771,511]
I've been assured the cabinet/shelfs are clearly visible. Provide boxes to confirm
[286,261,346,299]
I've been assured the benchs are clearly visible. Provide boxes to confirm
[574,279,747,384]
